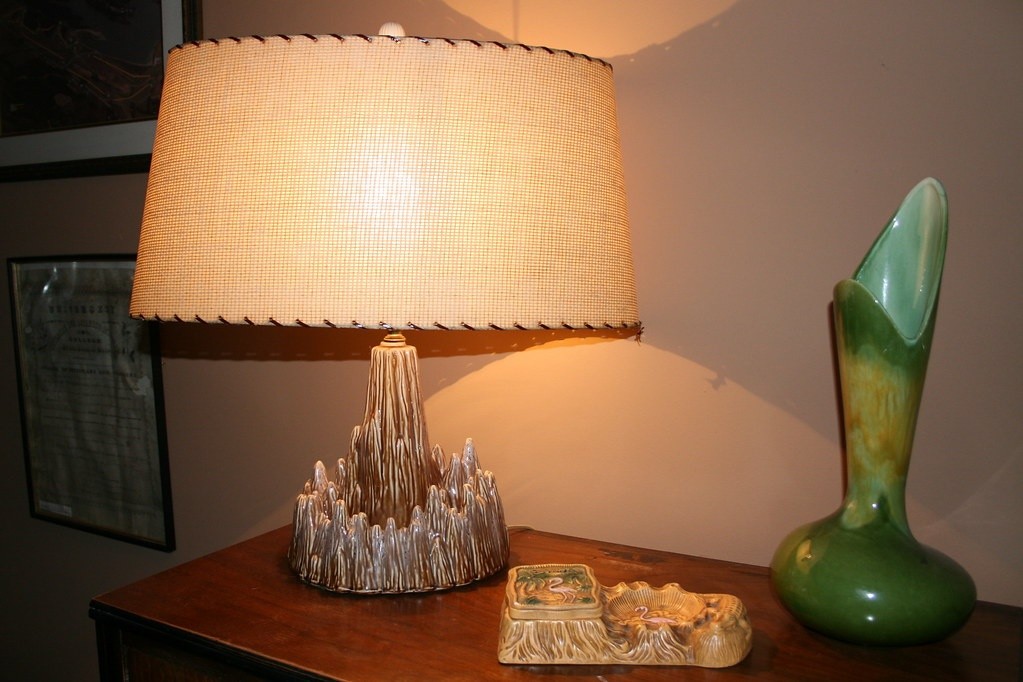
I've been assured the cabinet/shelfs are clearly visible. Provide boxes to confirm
[86,522,1023,682]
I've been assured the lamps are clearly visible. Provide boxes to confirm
[126,22,643,596]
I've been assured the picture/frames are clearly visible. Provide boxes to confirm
[0,0,204,183]
